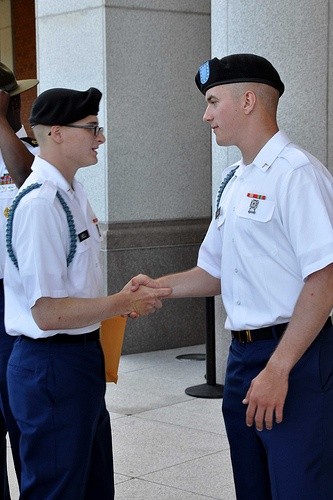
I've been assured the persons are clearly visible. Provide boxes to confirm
[0,62,39,500]
[4,86,172,500]
[120,53,333,500]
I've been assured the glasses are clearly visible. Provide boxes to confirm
[48,124,103,137]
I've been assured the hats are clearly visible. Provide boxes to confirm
[29,87,102,125]
[195,54,285,98]
[0,62,39,97]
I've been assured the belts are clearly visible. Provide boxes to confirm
[231,317,332,343]
